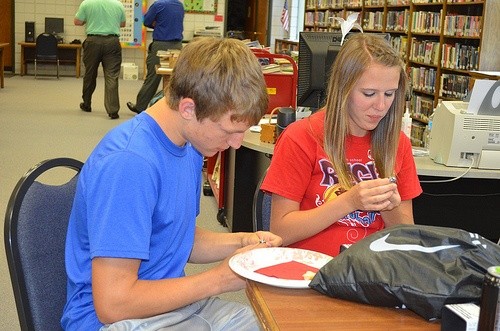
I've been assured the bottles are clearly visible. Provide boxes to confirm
[425,108,436,151]
[401,108,412,139]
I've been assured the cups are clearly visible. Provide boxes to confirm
[169,55,177,69]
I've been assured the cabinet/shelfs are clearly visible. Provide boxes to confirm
[305,0,487,147]
[273,39,299,55]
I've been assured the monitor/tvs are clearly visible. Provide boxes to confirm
[298,31,390,110]
[45,17,64,34]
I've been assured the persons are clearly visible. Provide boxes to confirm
[60,37,283,331]
[127,0,184,114]
[260,33,422,257]
[74,0,126,119]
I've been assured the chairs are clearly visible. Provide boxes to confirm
[4,155,87,331]
[35,32,60,79]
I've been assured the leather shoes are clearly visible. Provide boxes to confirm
[127,102,141,114]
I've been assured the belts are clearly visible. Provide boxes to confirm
[88,34,119,37]
[152,39,182,42]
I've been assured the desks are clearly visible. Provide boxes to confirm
[153,64,294,192]
[18,41,83,77]
[233,244,500,330]
[0,42,11,87]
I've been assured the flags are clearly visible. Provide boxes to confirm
[281,0,288,32]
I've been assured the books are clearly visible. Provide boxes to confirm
[159,48,180,67]
[409,67,437,93]
[444,16,482,36]
[244,39,293,72]
[364,11,383,30]
[410,38,440,65]
[411,10,443,33]
[305,11,362,32]
[307,0,481,9]
[411,95,443,146]
[389,36,408,58]
[387,9,409,31]
[440,74,471,100]
[441,42,480,71]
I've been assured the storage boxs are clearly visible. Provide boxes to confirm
[120,62,139,82]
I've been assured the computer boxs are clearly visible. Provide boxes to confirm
[25,22,34,43]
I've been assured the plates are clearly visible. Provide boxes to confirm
[228,247,334,288]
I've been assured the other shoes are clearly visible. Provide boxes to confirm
[80,103,91,112]
[110,113,120,119]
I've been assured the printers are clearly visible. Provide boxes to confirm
[429,101,500,170]
